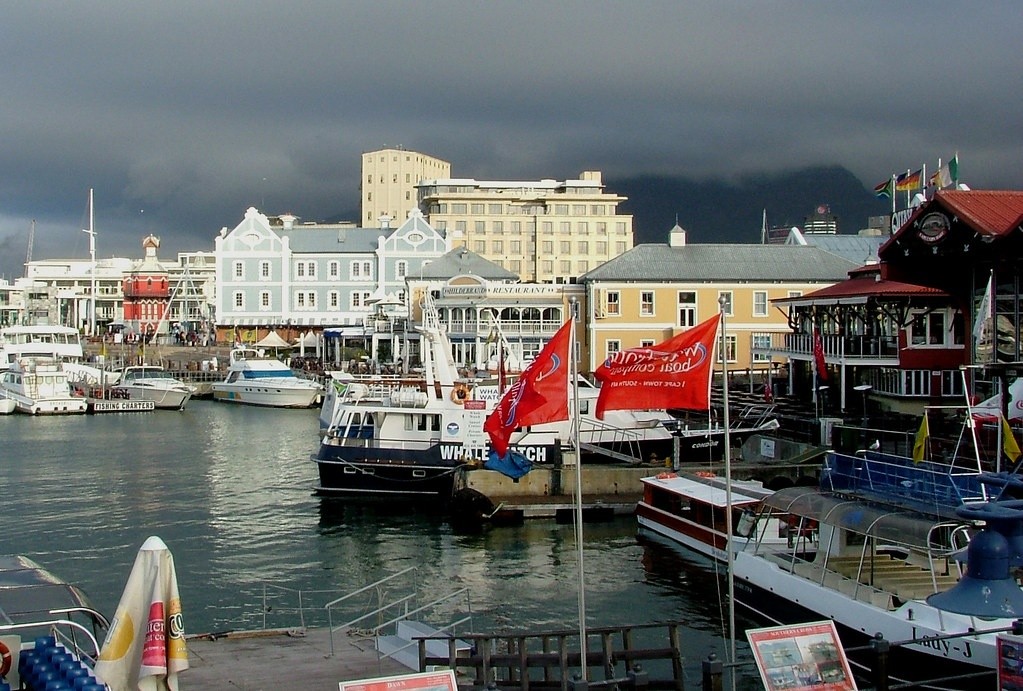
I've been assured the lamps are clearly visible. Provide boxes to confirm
[926,520,1023,621]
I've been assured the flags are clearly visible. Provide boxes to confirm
[498,346,506,397]
[594,313,719,421]
[814,324,828,380]
[913,414,929,466]
[483,318,572,459]
[1002,414,1021,463]
[896,168,922,191]
[895,172,908,191]
[941,156,959,188]
[972,276,992,340]
[922,169,940,189]
[764,380,771,404]
[874,178,892,199]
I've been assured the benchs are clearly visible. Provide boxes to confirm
[824,554,959,605]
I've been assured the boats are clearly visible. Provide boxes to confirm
[112,335,197,410]
[212,349,323,408]
[0,356,88,415]
[0,324,121,387]
[635,449,1023,681]
[310,288,781,499]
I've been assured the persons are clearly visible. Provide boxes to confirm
[103,328,159,345]
[71,384,130,400]
[173,326,217,347]
[290,355,420,376]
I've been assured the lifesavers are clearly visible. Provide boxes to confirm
[789,513,818,536]
[452,386,470,405]
[656,473,677,479]
[695,471,716,477]
